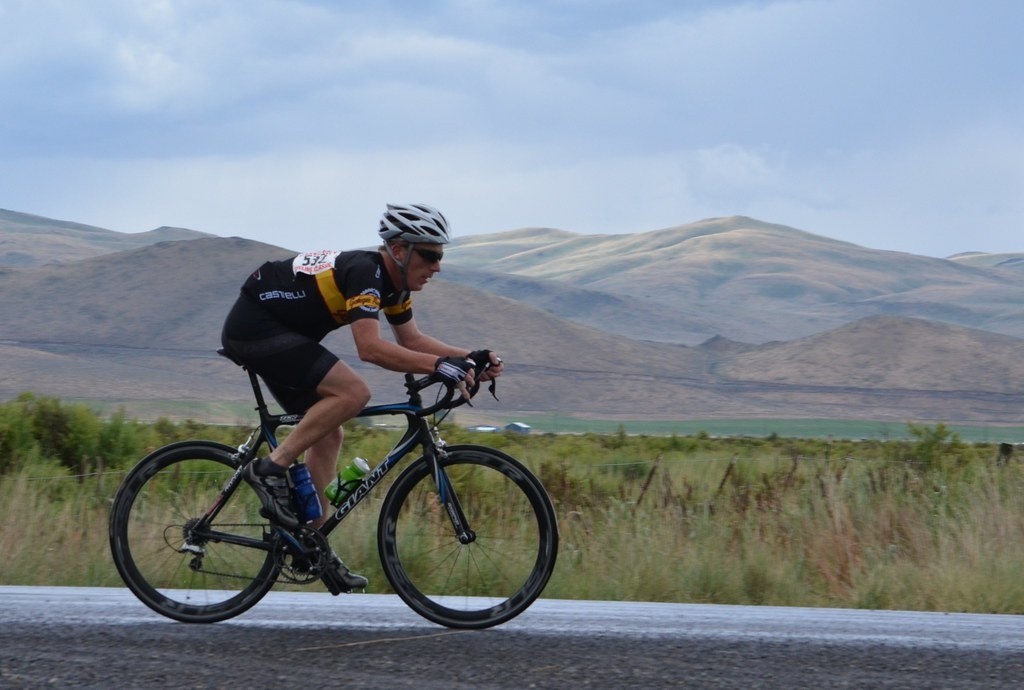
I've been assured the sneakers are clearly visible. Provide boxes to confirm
[241,457,299,528]
[291,538,369,588]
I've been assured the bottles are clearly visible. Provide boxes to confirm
[289,456,371,521]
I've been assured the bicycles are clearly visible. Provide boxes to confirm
[110,348,560,629]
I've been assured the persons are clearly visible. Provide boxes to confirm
[220,202,504,589]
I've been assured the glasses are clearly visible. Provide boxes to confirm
[402,244,443,264]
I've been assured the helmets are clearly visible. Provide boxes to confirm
[379,201,450,244]
